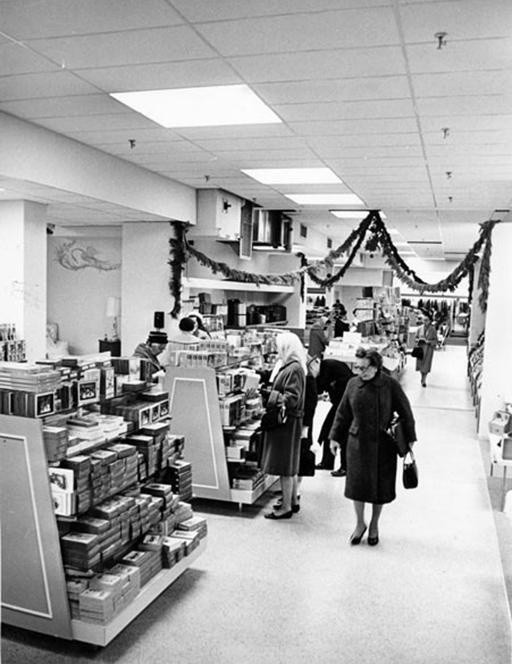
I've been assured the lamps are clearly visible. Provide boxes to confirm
[105,297,120,341]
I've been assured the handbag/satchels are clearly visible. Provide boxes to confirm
[402,452,418,489]
[260,407,289,432]
[411,344,424,360]
[384,416,410,458]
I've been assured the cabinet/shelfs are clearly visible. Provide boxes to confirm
[1,340,281,652]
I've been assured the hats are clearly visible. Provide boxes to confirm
[148,331,168,344]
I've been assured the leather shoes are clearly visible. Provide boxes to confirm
[265,509,293,519]
[351,526,367,545]
[331,467,347,477]
[273,504,300,513]
[315,462,334,470]
[367,531,378,545]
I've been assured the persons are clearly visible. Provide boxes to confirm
[440,307,450,347]
[413,313,439,388]
[334,311,355,337]
[131,329,166,384]
[306,352,357,476]
[327,345,418,546]
[307,315,330,359]
[187,311,211,339]
[249,330,307,519]
[333,298,346,316]
[420,305,438,322]
[239,363,320,512]
[173,316,199,342]
